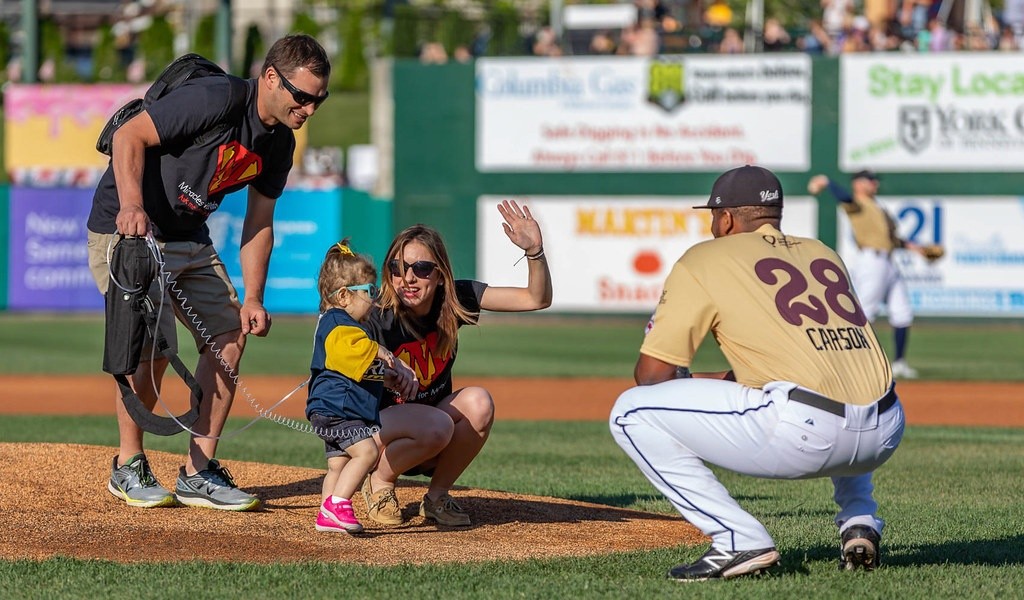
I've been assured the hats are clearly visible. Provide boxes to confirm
[692,165,783,208]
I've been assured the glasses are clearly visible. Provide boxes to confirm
[271,64,328,107]
[388,260,439,277]
[329,283,378,299]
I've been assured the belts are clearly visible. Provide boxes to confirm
[789,385,897,417]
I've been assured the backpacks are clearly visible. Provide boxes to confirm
[96,52,245,157]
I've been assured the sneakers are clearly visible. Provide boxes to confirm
[317,512,365,537]
[108,452,183,508]
[668,546,782,582]
[838,525,880,571]
[321,495,362,533]
[419,494,471,527]
[362,475,404,523]
[172,458,263,511]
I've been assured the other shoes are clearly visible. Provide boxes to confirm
[891,358,918,380]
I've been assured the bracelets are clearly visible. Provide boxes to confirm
[515,245,545,266]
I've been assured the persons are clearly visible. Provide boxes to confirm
[58,36,330,509]
[807,169,945,380]
[306,239,397,532]
[0,0,1024,82]
[608,165,905,582]
[366,200,553,526]
